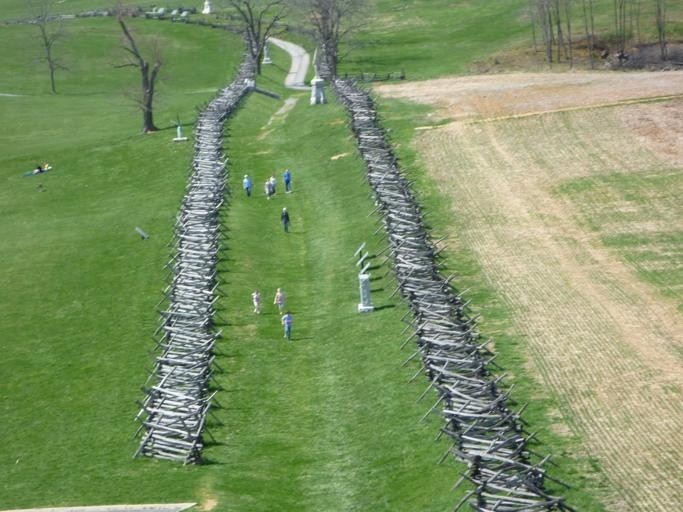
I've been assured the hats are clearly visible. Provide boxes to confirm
[244,175,248,178]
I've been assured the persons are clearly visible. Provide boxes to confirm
[22,162,52,178]
[281,311,294,339]
[265,175,276,200]
[242,174,252,197]
[273,287,285,314]
[251,288,261,314]
[280,207,289,233]
[282,169,292,194]
